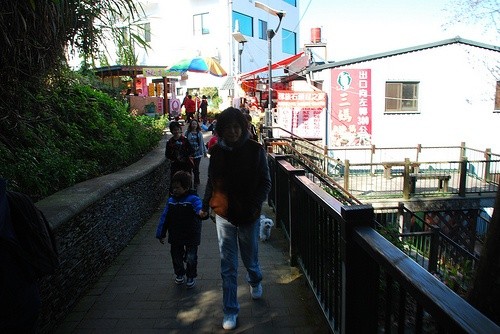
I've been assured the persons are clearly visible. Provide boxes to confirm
[155,92,258,289]
[0,178,40,334]
[199,107,271,330]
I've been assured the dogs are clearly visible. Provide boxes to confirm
[260,214,274,242]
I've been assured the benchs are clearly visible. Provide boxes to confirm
[410,173,451,193]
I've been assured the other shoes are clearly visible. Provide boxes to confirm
[222,311,238,330]
[175,275,185,286]
[250,282,263,300]
[186,277,195,289]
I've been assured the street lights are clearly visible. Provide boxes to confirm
[254,0,286,153]
[232,32,249,107]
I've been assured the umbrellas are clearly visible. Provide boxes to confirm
[166,54,227,77]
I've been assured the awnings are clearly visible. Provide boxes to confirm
[237,52,305,82]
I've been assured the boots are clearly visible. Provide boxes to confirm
[193,171,200,187]
